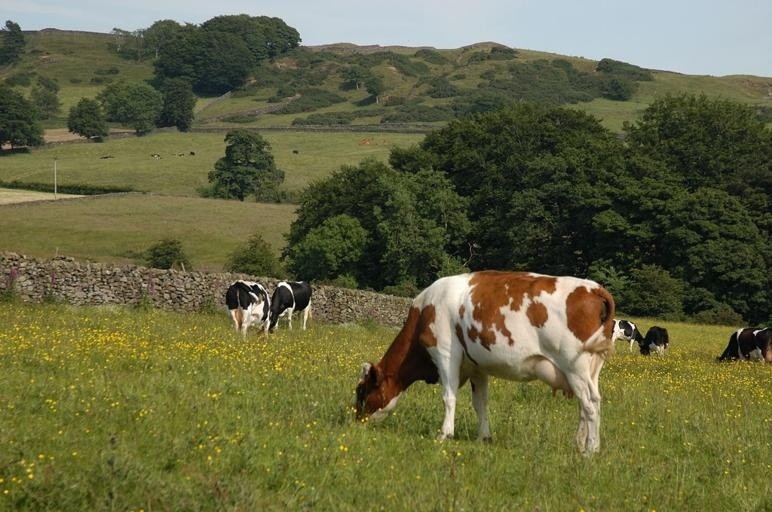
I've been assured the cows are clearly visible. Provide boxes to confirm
[271,279,315,331]
[352,267,617,456]
[641,325,670,356]
[612,318,641,353]
[223,278,273,339]
[714,325,771,365]
